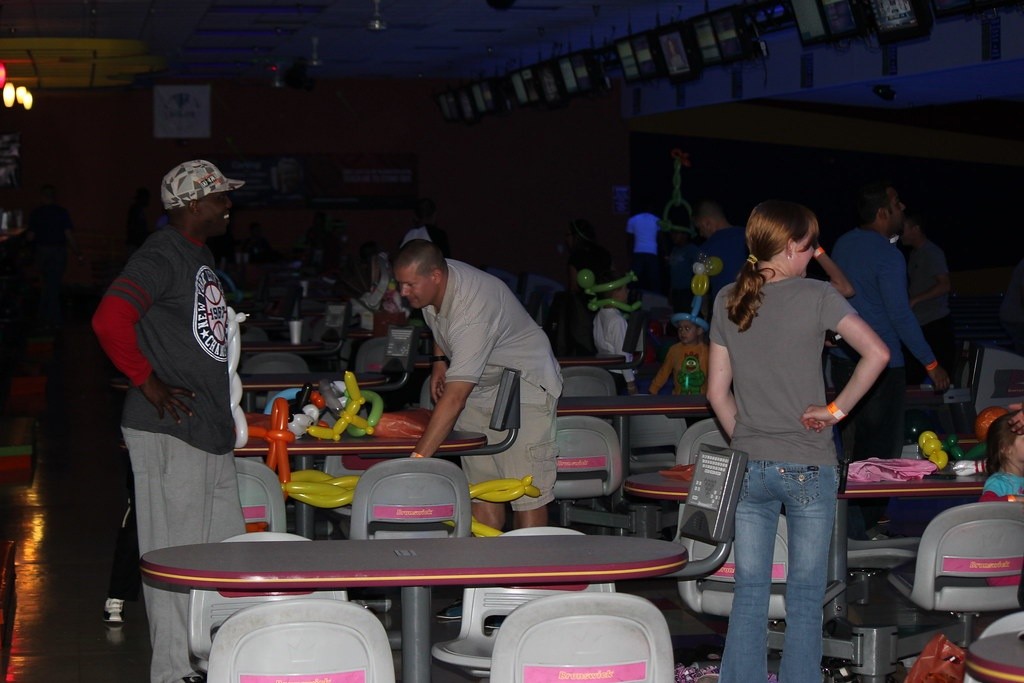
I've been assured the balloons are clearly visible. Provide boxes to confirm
[919,406,1008,470]
[225,306,542,537]
[671,257,721,333]
[578,270,640,319]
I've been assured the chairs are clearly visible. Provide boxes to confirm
[186,531,349,671]
[675,418,787,618]
[234,457,287,534]
[629,414,686,470]
[489,593,675,683]
[241,352,310,409]
[951,342,985,434]
[349,457,472,540]
[431,526,616,679]
[552,416,621,504]
[356,336,391,372]
[560,367,616,397]
[241,326,269,342]
[207,599,396,683]
[886,502,1024,613]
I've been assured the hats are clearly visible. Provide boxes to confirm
[160,159,245,210]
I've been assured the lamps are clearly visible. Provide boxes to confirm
[304,36,323,67]
[267,63,285,88]
[367,0,388,32]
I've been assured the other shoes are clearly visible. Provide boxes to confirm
[863,525,891,541]
[102,598,129,623]
[875,514,892,523]
[435,599,463,619]
[821,616,851,677]
[174,674,206,683]
[484,614,507,629]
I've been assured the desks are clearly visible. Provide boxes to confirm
[346,329,432,338]
[233,431,489,539]
[315,297,344,304]
[555,354,626,366]
[414,354,432,368]
[766,473,988,683]
[827,384,929,459]
[301,310,325,316]
[145,534,689,683]
[246,320,283,327]
[241,371,390,413]
[557,396,710,537]
[241,341,324,353]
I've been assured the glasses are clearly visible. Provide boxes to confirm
[565,232,574,238]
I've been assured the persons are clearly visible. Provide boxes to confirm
[827,185,954,524]
[999,259,1024,356]
[25,184,76,331]
[668,40,683,68]
[92,159,245,683]
[545,198,750,395]
[707,199,891,683]
[304,213,344,270]
[394,239,564,629]
[349,208,451,316]
[980,399,1024,610]
[213,232,235,270]
[241,221,272,263]
[803,242,869,540]
[125,187,152,255]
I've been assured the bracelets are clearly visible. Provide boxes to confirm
[429,356,450,368]
[826,402,848,420]
[926,361,937,370]
[410,452,423,458]
[1008,494,1016,502]
[813,247,824,258]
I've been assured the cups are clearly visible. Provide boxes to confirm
[288,321,304,346]
